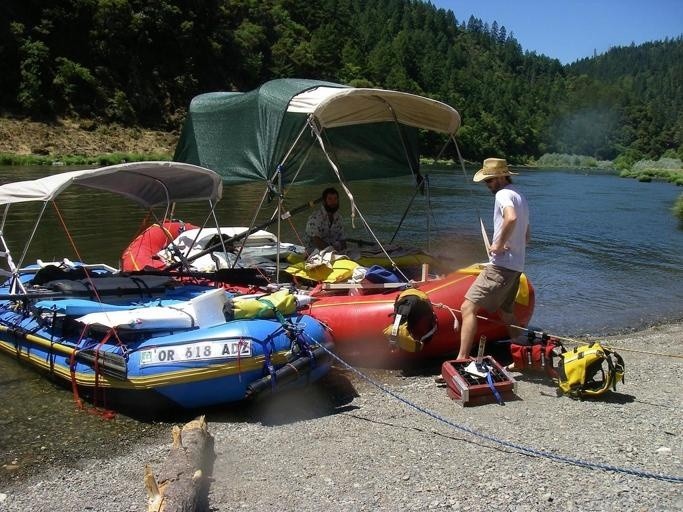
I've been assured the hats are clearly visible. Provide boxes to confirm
[473,158,518,182]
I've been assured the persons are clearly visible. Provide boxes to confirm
[302,187,346,258]
[434,158,531,384]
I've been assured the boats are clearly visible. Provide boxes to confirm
[120,76,536,371]
[0,160,335,410]
